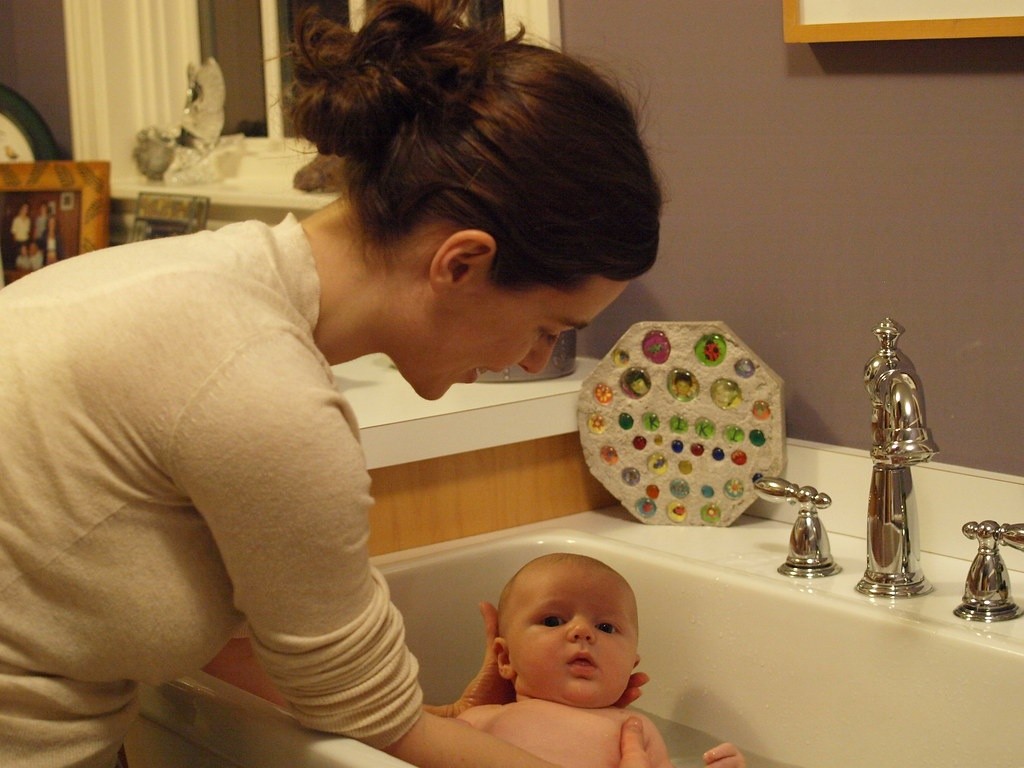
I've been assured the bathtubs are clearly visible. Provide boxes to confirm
[211,502,1024,768]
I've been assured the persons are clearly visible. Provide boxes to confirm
[456,553,747,768]
[0,0,662,768]
[9,201,62,273]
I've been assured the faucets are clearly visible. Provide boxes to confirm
[852,315,941,598]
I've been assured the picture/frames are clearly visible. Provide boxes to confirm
[132,190,211,244]
[0,161,112,285]
